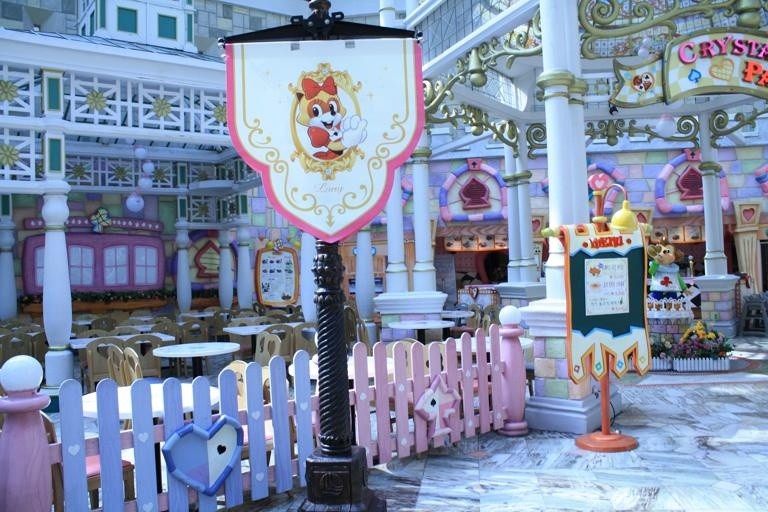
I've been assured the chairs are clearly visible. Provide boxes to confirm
[2,303,504,512]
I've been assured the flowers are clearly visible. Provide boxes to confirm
[649,321,738,360]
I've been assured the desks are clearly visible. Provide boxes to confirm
[222,322,316,355]
[387,320,456,345]
[441,332,533,359]
[66,332,178,350]
[439,310,475,326]
[75,381,219,496]
[149,341,243,381]
[287,353,396,445]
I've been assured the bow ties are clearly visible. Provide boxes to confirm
[329,126,339,135]
